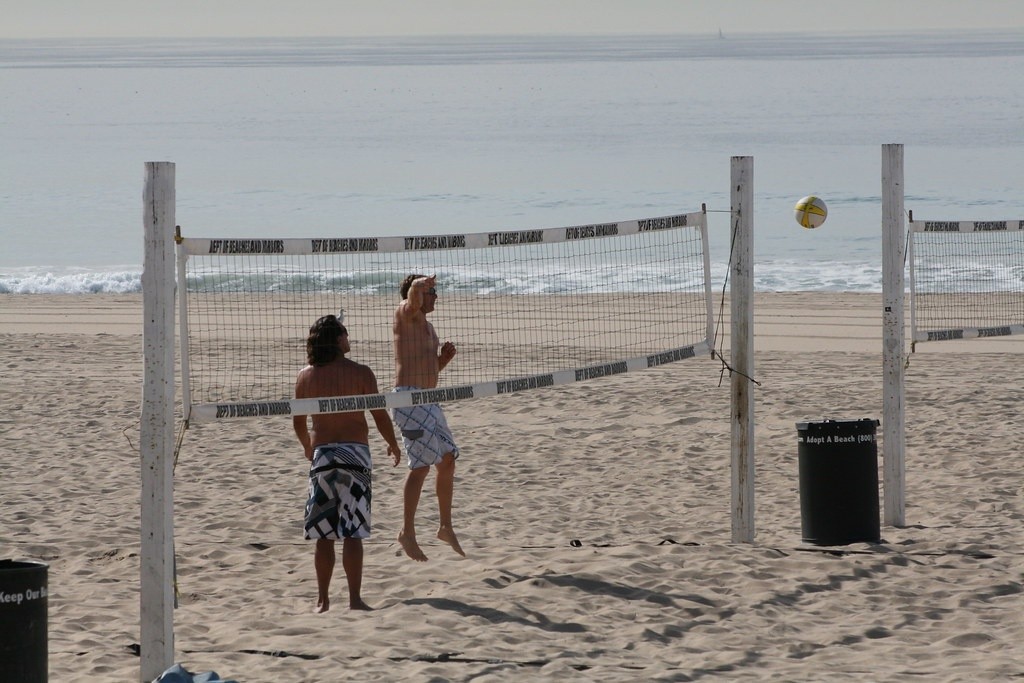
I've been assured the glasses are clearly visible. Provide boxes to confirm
[428,287,435,295]
[342,327,348,336]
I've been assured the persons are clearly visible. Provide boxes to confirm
[392,275,465,562]
[293,314,401,614]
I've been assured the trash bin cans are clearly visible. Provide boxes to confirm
[0,557,51,683]
[795,417,883,546]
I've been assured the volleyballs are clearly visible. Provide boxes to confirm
[793,195,828,230]
